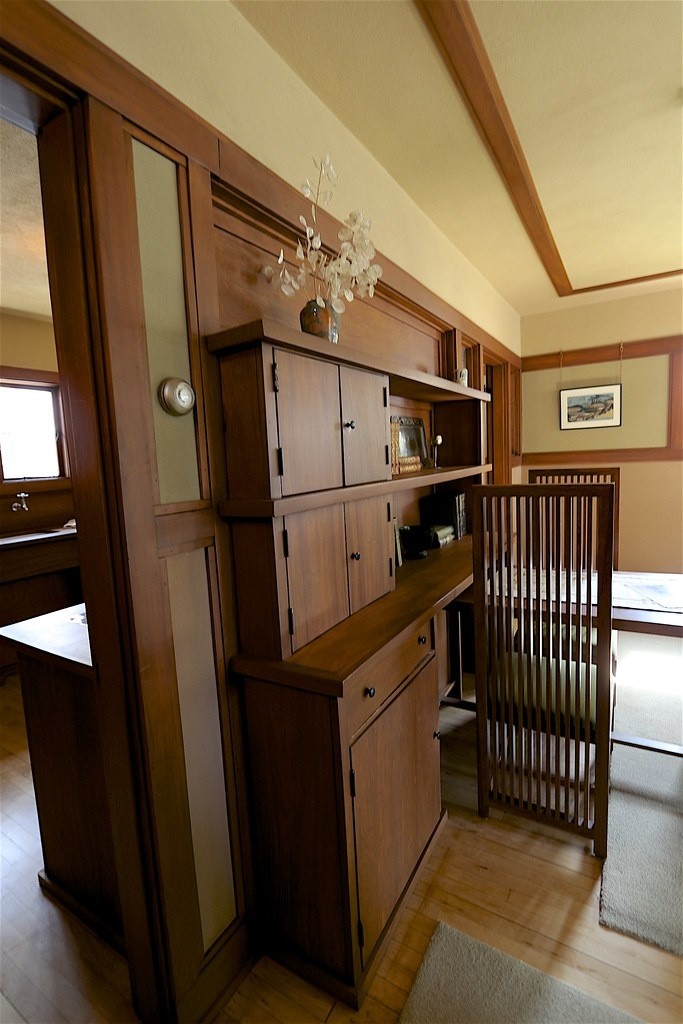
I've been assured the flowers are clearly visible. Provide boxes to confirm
[264,151,383,313]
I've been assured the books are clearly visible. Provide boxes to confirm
[393,491,467,568]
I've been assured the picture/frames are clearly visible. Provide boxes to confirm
[390,415,428,472]
[560,383,622,430]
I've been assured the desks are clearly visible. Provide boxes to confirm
[0,603,121,938]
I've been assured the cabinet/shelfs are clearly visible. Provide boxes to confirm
[205,314,516,1013]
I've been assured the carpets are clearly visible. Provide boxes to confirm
[600,631,683,958]
[396,919,646,1023]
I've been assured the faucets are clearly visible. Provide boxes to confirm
[11,492,29,512]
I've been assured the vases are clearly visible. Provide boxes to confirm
[300,298,339,343]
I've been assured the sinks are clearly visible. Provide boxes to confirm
[0,529,60,540]
